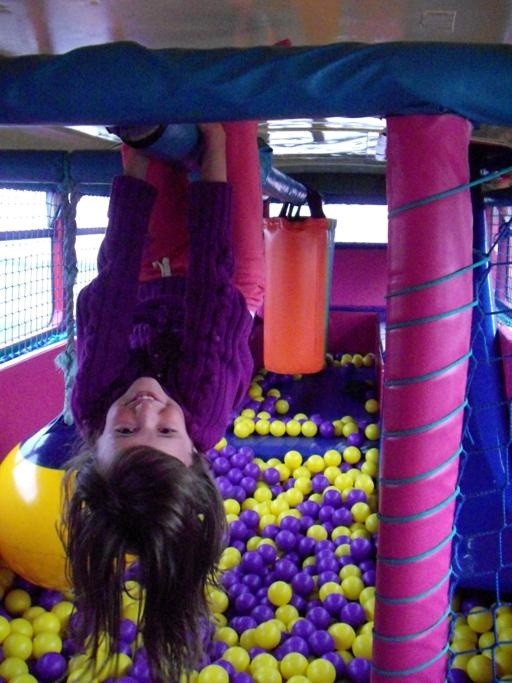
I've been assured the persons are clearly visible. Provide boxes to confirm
[53,121,269,682]
